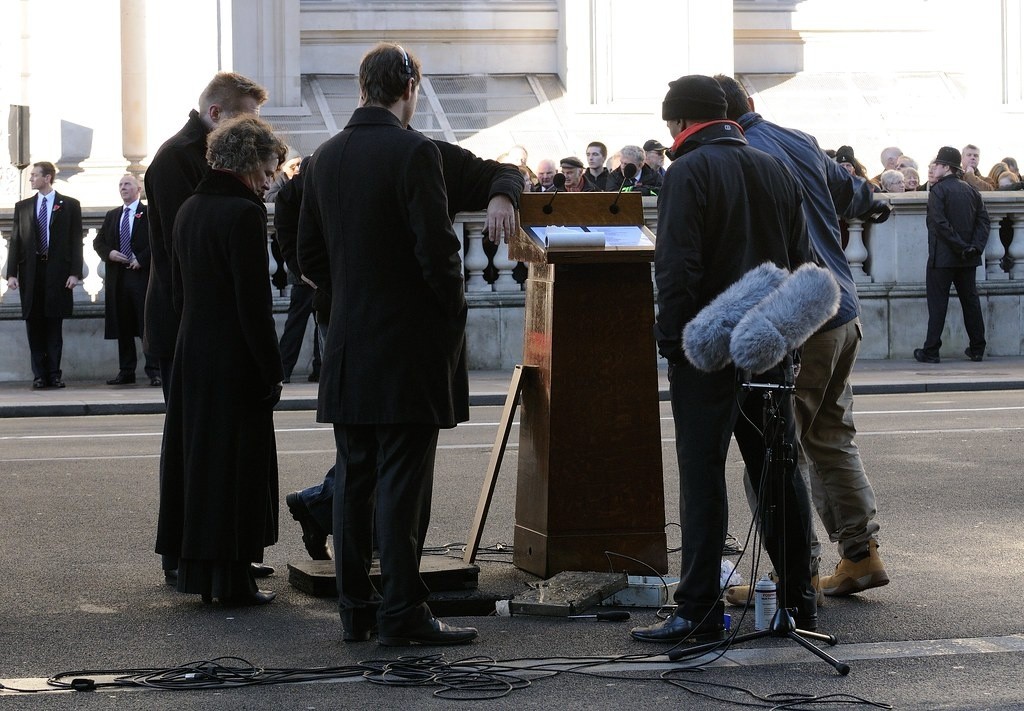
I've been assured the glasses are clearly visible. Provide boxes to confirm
[294,162,300,167]
[650,152,663,156]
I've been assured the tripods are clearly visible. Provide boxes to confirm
[671,357,850,676]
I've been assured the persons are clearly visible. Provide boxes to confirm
[585,143,610,191]
[915,146,989,364]
[93,174,163,386]
[264,41,533,644]
[867,143,1024,192]
[558,156,605,193]
[607,139,672,193]
[7,162,83,391]
[144,69,291,606]
[628,71,894,643]
[530,159,559,193]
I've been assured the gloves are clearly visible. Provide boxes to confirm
[962,247,978,261]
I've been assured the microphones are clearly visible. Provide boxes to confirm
[610,163,636,214]
[729,263,842,373]
[683,260,791,372]
[543,173,566,213]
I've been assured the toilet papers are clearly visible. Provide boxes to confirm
[544,223,606,247]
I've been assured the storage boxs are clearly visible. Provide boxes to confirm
[602,573,682,609]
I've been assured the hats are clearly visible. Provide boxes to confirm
[836,155,856,169]
[560,156,586,169]
[661,75,728,121]
[932,147,962,170]
[643,140,669,152]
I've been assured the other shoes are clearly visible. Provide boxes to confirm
[33,376,65,389]
[286,489,333,561]
[202,588,278,604]
[107,370,136,384]
[164,569,179,584]
[965,347,983,361]
[250,563,274,578]
[150,376,161,385]
[914,348,941,363]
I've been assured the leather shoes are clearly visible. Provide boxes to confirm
[794,612,819,631]
[342,619,383,642]
[630,612,725,644]
[379,617,478,647]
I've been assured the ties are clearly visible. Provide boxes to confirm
[630,178,637,185]
[120,208,132,262]
[37,197,48,260]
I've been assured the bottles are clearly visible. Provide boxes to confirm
[754,573,777,630]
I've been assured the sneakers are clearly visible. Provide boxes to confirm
[819,539,890,597]
[726,570,825,607]
